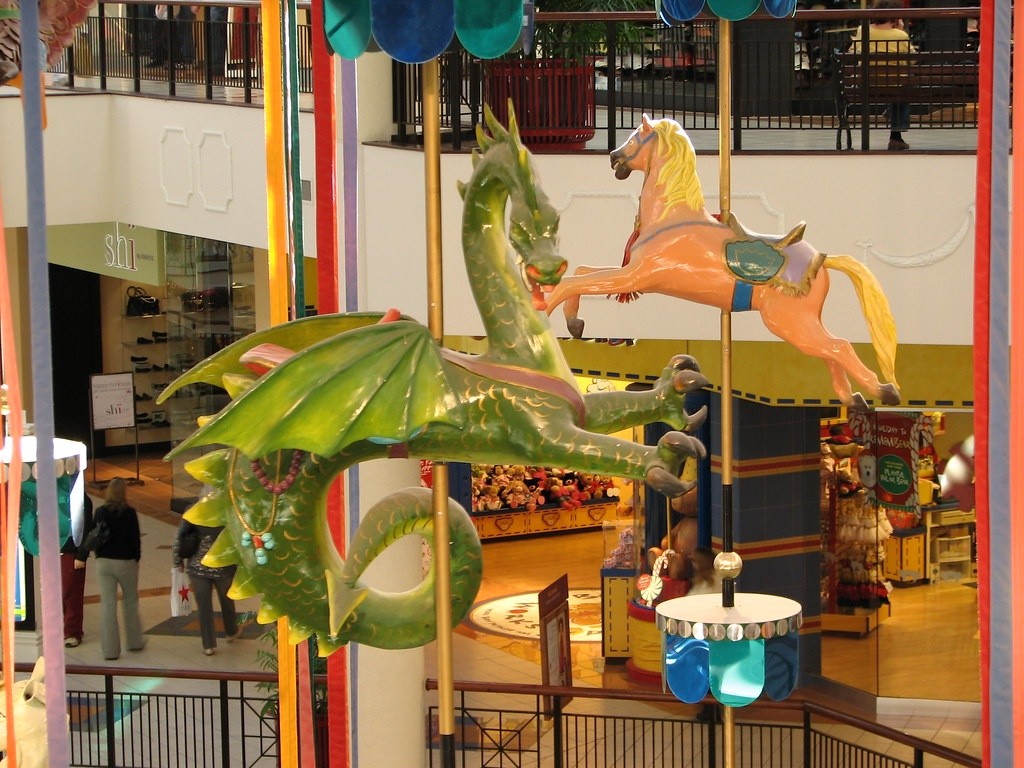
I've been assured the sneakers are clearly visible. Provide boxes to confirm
[65,637,79,648]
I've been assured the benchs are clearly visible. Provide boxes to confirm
[829,47,1013,151]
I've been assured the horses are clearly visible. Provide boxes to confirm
[541,112,901,407]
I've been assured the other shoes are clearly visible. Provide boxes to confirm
[226,628,241,642]
[130,331,194,429]
[202,647,213,655]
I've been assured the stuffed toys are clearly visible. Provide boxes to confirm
[603,528,634,569]
[470,463,620,513]
[819,442,894,610]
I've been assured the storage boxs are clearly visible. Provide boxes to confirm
[946,526,968,538]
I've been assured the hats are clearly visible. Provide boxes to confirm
[829,426,842,435]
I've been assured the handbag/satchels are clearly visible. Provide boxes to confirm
[82,521,109,552]
[125,285,159,316]
[181,287,233,313]
[170,566,192,617]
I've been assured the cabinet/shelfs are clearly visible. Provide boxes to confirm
[936,535,972,578]
[120,312,199,432]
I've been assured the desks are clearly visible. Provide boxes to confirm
[921,507,976,579]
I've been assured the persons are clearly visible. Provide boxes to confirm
[144,2,227,75]
[172,482,241,654]
[849,0,917,150]
[60,490,93,648]
[74,476,144,659]
